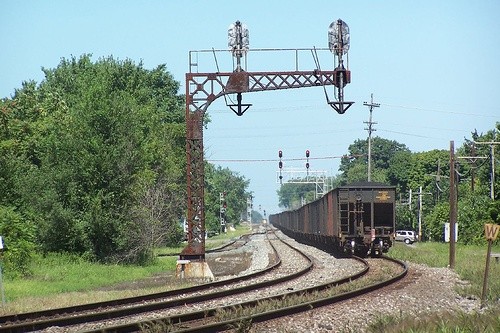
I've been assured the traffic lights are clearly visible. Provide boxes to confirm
[277,150,310,180]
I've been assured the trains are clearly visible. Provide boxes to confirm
[268,182,396,259]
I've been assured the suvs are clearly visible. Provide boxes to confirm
[395,230,417,245]
